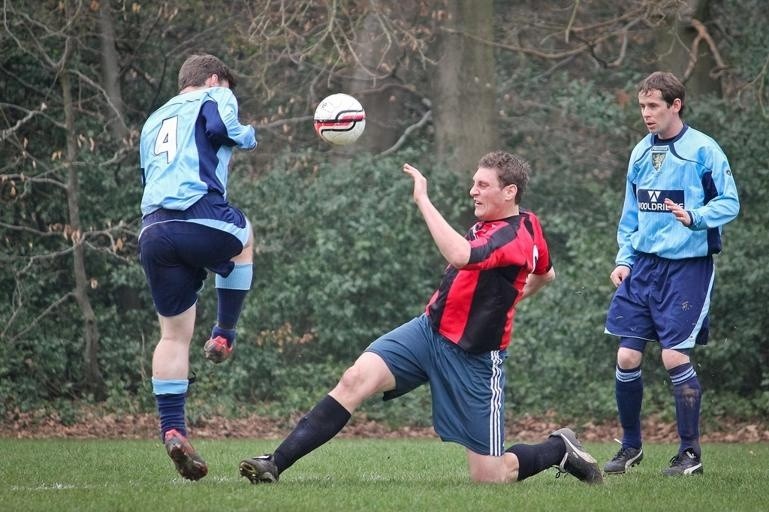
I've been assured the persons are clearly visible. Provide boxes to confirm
[604,71,741,475]
[138,53,258,482]
[238,150,605,487]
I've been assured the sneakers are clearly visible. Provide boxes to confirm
[661,446,704,478]
[164,428,210,482]
[603,445,644,475]
[545,424,604,486]
[202,326,237,364]
[238,453,281,485]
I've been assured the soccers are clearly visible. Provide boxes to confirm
[313,93,366,145]
